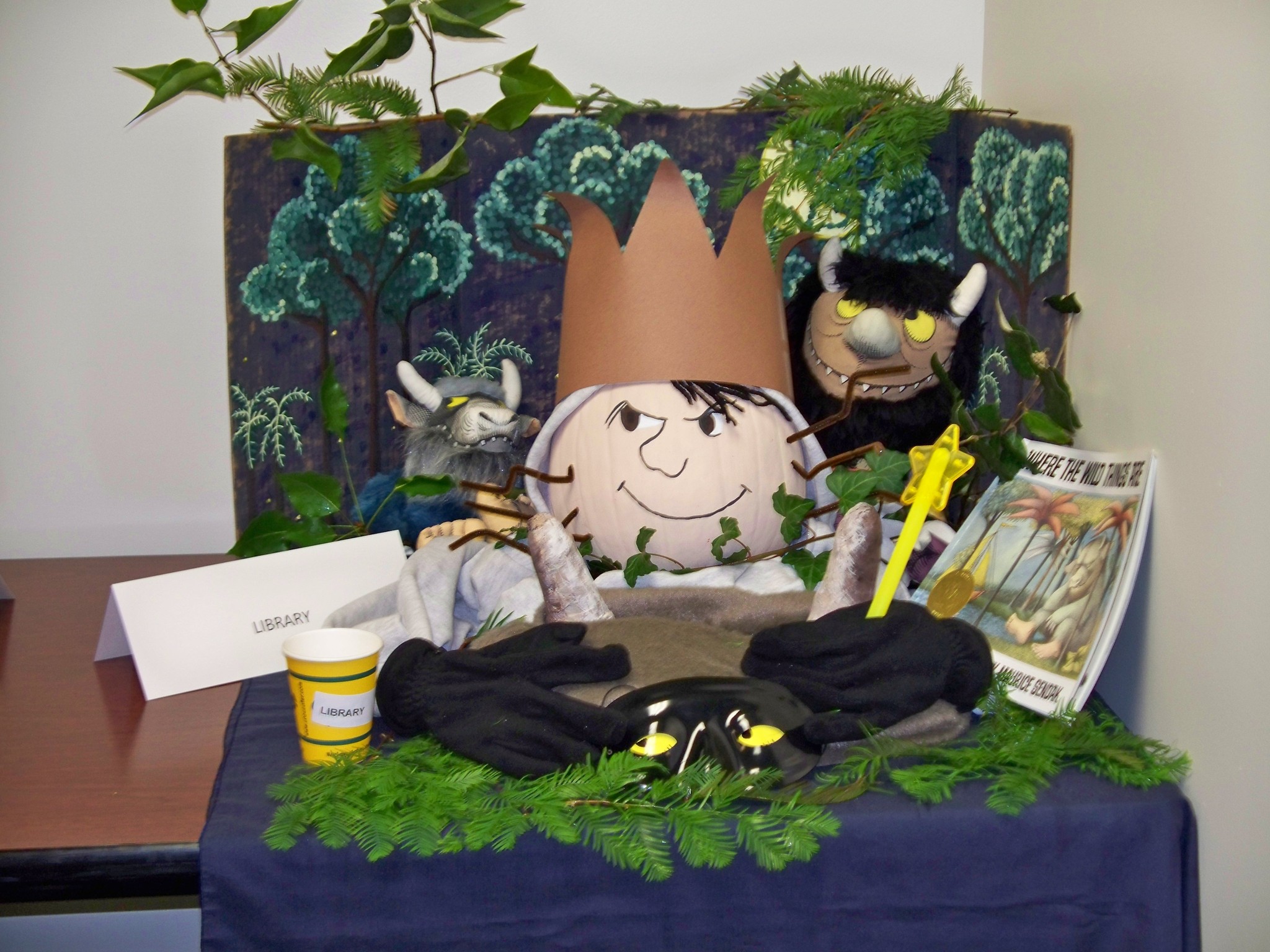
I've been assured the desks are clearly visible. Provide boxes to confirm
[0,552,1201,952]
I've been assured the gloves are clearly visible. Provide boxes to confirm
[376,639,629,784]
[736,597,996,745]
[467,623,635,691]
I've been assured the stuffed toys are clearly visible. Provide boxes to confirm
[776,241,986,457]
[382,357,537,488]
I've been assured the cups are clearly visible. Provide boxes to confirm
[281,627,384,767]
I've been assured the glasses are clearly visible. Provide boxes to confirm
[600,676,826,788]
